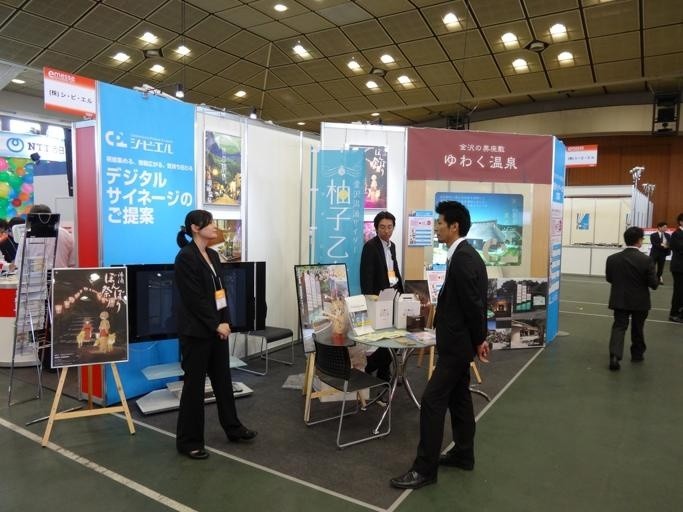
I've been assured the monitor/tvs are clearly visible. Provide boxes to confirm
[109,260,267,344]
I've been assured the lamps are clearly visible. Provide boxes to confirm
[175,82,183,99]
[247,110,257,120]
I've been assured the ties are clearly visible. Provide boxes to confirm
[660,231,663,244]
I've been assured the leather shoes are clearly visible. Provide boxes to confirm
[611,355,620,370]
[633,355,645,362]
[390,469,438,489]
[229,428,257,443]
[659,281,664,285]
[180,447,210,459]
[670,315,683,323]
[438,452,474,471]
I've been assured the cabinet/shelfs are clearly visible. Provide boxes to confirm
[0,269,39,367]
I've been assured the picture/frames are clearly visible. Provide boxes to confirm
[295,262,357,354]
[203,129,241,207]
[210,219,243,262]
[49,267,129,370]
[352,144,388,209]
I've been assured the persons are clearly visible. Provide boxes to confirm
[669,213,683,323]
[605,227,662,370]
[15,204,76,374]
[173,209,259,461]
[648,221,671,285]
[360,210,405,383]
[388,201,490,489]
[2,217,26,264]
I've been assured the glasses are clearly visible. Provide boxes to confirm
[378,225,393,232]
[641,237,645,240]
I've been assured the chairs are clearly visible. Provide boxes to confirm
[231,325,295,376]
[303,333,392,449]
